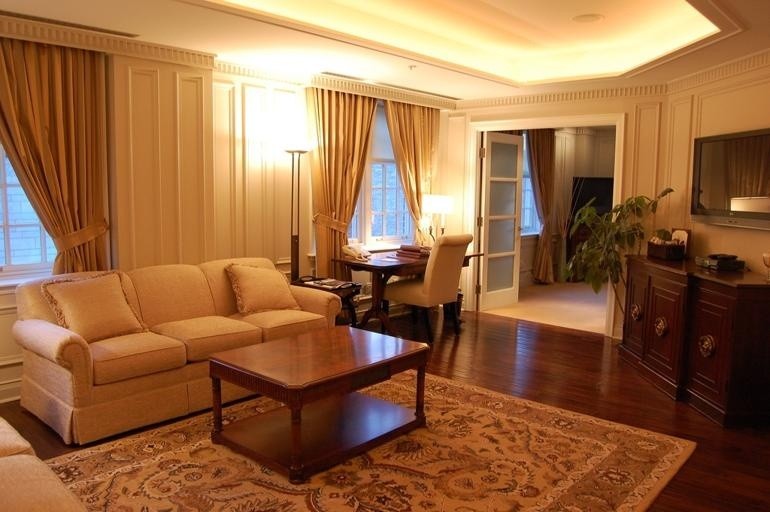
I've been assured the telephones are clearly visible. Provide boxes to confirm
[342,245,372,258]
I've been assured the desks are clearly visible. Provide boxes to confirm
[329,249,484,334]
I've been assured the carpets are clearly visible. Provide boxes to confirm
[40,368,699,512]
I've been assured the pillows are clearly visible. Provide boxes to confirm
[38,266,145,347]
[223,261,301,316]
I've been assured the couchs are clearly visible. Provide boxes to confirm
[11,257,343,450]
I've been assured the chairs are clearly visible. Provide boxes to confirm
[378,234,473,343]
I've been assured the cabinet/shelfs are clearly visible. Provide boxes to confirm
[616,253,770,430]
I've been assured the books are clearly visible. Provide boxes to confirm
[396,245,431,259]
